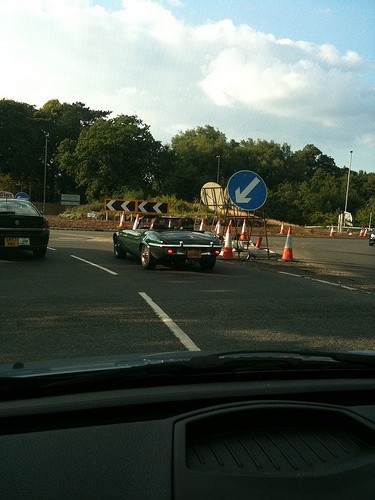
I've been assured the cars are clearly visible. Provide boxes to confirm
[0,196,50,258]
[113,213,222,271]
[369,230,375,245]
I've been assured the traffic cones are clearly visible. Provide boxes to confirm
[199,218,205,231]
[280,221,285,234]
[132,214,140,230]
[363,227,367,237]
[359,227,364,236]
[223,220,233,257]
[240,216,246,240]
[118,211,126,228]
[329,225,334,237]
[215,220,221,237]
[281,226,293,260]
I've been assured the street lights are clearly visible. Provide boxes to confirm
[43,133,50,206]
[217,156,220,183]
[344,149,353,212]
